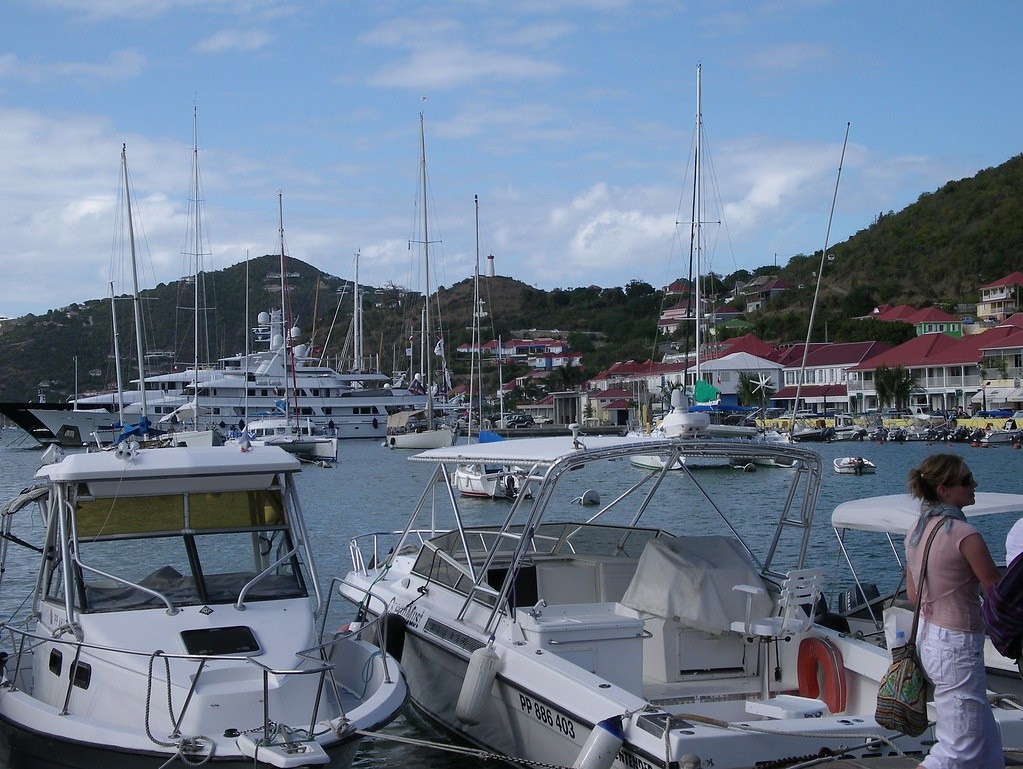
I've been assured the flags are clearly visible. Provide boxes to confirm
[443,362,454,390]
[434,338,445,358]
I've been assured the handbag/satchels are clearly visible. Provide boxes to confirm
[980,550,1023,659]
[875,643,928,737]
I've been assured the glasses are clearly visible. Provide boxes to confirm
[946,472,974,487]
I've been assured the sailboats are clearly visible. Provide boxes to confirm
[449,191,535,504]
[380,112,465,451]
[616,57,779,473]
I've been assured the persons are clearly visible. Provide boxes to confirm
[905,454,1005,769]
[465,413,495,430]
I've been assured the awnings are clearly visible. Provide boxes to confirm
[971,387,1018,404]
[1007,388,1023,403]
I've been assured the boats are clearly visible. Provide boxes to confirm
[320,410,1023,769]
[833,455,878,474]
[805,489,1023,706]
[0,138,416,769]
[786,414,981,443]
[977,416,1023,445]
[1,103,399,467]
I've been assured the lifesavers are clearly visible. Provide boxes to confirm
[796,636,848,713]
